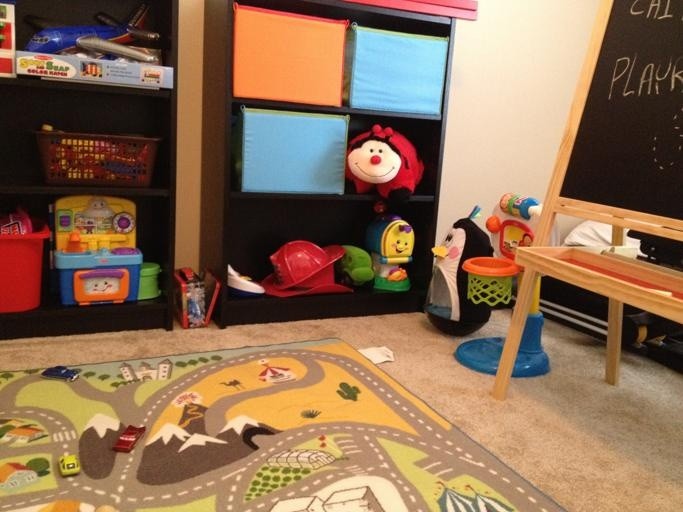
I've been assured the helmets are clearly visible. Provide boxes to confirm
[271,240,346,290]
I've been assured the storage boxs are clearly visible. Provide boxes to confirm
[0,221,51,314]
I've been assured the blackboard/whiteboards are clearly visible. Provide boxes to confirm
[554,0,683,233]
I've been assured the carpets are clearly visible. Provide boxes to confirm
[0,339,567,512]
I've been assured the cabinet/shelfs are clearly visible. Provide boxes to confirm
[199,0,455,329]
[0,0,178,340]
[492,1,683,401]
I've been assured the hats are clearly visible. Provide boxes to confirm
[259,263,353,298]
